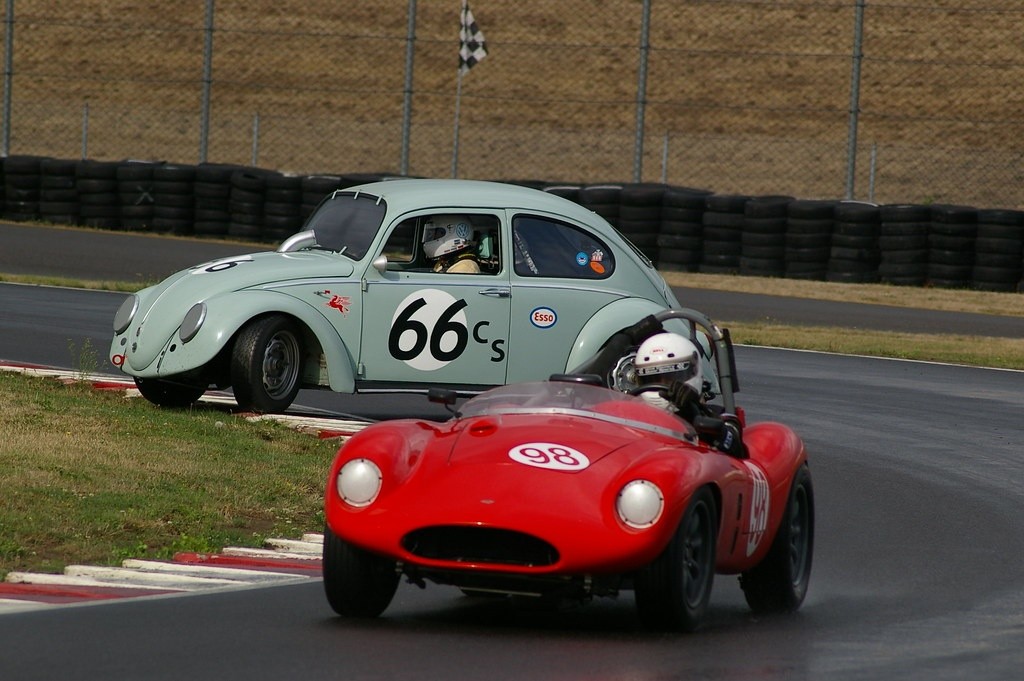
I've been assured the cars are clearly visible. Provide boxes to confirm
[105,181,719,415]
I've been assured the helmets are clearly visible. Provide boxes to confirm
[634,333,704,397]
[422,213,474,260]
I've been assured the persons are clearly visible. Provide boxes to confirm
[422,214,482,274]
[634,333,743,456]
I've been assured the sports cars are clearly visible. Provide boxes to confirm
[322,373,815,637]
